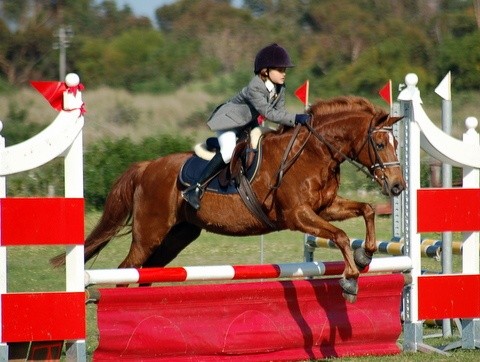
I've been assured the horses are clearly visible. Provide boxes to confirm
[48,95,409,304]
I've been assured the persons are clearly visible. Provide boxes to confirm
[182,42,309,211]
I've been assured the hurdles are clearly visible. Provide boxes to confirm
[0,72,480,361]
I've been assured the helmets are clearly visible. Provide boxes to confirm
[254,43,296,68]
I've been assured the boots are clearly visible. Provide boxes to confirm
[185,152,227,210]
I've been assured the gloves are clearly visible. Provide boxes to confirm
[295,114,309,125]
